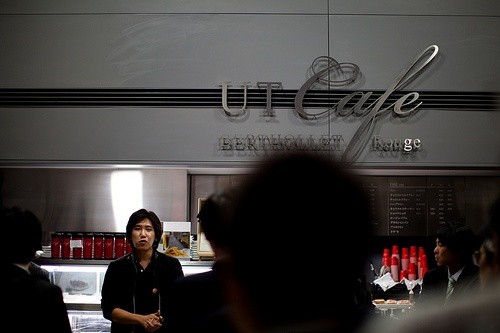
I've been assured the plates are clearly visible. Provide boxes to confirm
[372,300,412,310]
[160,250,192,259]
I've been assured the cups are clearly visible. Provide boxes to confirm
[382,244,427,281]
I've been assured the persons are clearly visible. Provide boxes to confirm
[422,220,481,299]
[161,187,243,333]
[216,144,398,333]
[0,205,73,333]
[417,192,500,333]
[101,209,185,333]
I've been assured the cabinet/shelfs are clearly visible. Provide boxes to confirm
[36,256,216,313]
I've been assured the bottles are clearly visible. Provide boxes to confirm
[191,234,199,261]
[71,233,131,259]
[50,232,61,258]
[60,233,71,260]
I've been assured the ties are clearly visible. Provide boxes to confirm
[444,277,457,302]
